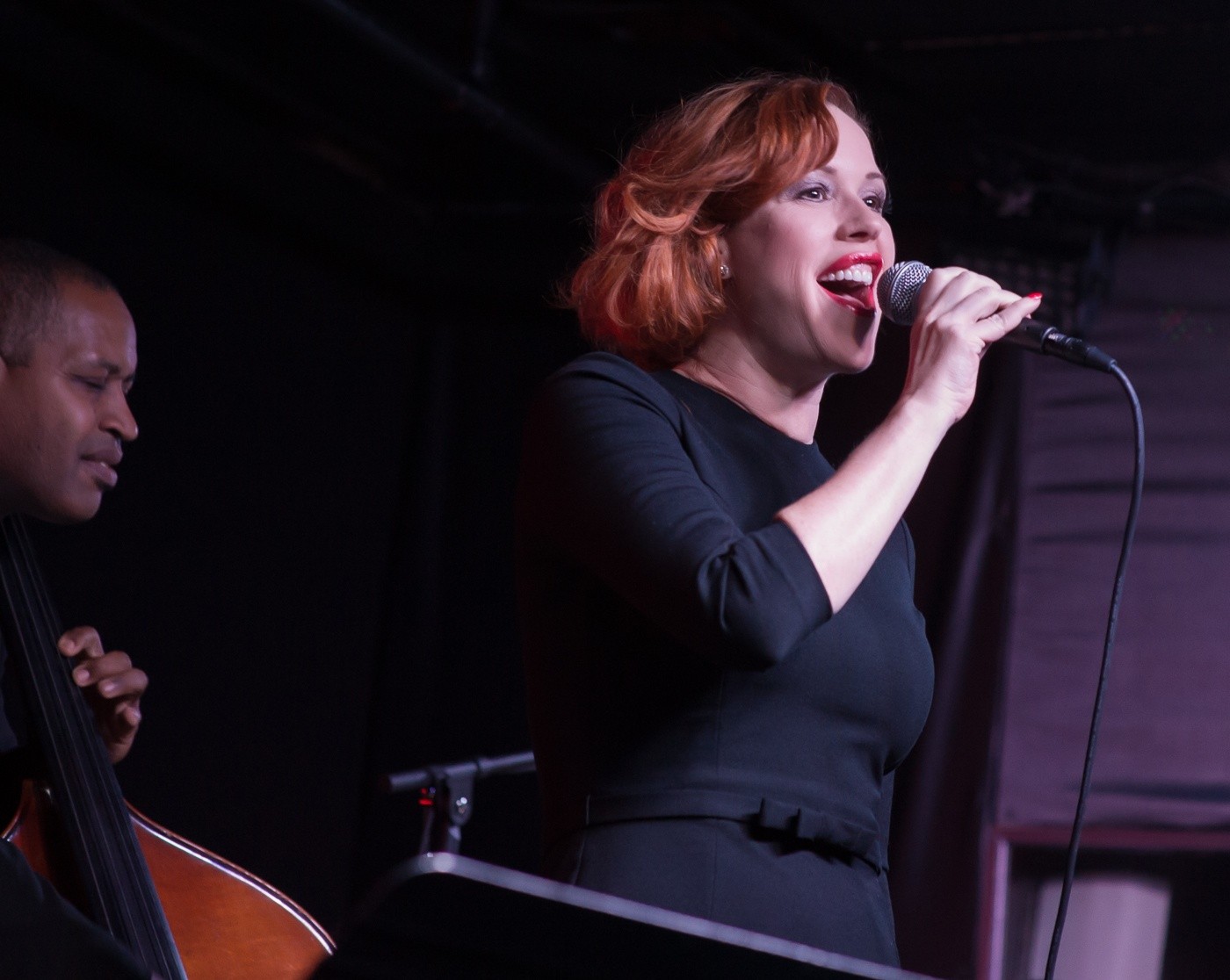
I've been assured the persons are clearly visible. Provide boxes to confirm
[1,246,147,980]
[483,78,1041,980]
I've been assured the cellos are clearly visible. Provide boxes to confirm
[3,518,342,980]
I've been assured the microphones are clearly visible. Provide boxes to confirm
[876,258,1121,377]
